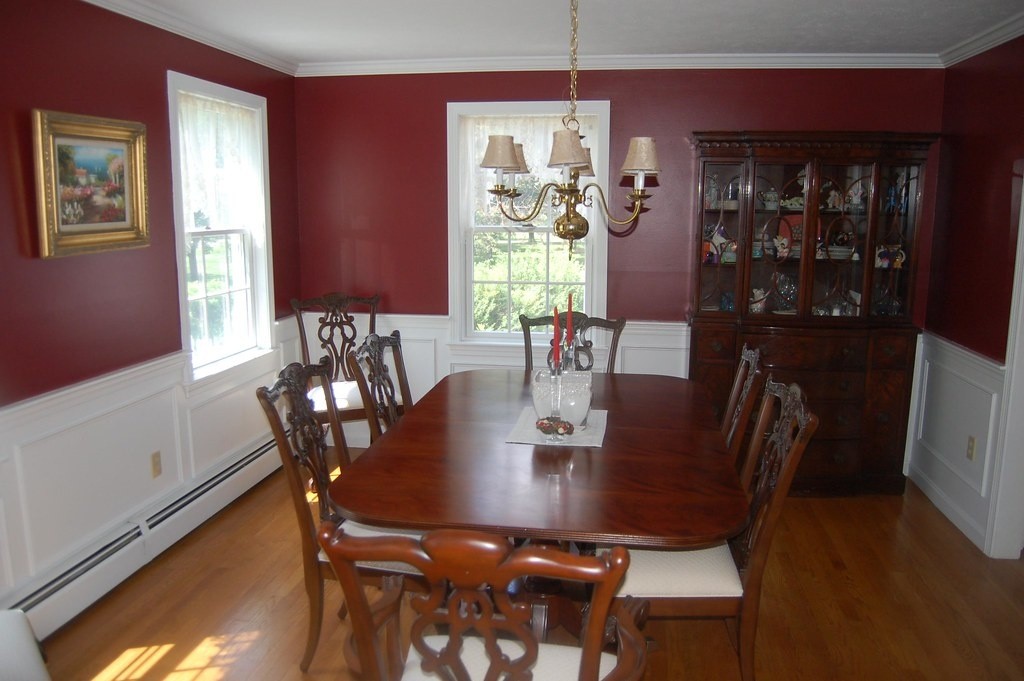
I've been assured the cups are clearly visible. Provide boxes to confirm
[757,186,779,210]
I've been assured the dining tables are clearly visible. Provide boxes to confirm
[327,369,748,652]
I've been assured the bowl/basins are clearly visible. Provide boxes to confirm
[531,369,592,426]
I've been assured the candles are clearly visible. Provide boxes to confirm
[567,291,574,347]
[553,305,560,370]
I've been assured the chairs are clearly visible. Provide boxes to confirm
[348,329,414,444]
[518,312,627,377]
[316,521,651,681]
[256,354,451,673]
[718,336,774,462]
[578,371,820,681]
[288,291,381,493]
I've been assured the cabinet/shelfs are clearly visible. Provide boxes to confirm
[687,130,942,324]
[690,318,922,497]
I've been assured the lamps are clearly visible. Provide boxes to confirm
[478,2,663,264]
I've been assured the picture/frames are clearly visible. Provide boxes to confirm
[32,107,151,259]
